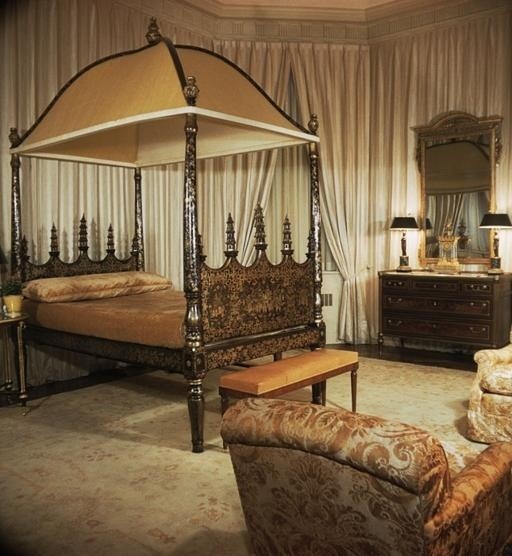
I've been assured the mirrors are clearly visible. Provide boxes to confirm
[409,109,503,274]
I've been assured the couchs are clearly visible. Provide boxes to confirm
[461,323,511,445]
[218,393,512,554]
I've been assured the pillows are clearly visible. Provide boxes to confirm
[21,264,174,305]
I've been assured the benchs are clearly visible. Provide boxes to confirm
[217,346,360,454]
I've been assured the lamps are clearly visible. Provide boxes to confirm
[388,215,420,273]
[476,211,512,275]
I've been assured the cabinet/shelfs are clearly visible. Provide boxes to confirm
[374,265,511,368]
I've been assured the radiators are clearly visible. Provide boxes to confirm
[312,268,346,346]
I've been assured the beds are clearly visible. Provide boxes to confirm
[9,12,329,457]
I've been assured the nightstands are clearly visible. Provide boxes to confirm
[0,307,34,417]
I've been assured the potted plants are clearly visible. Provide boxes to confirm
[2,274,24,312]
